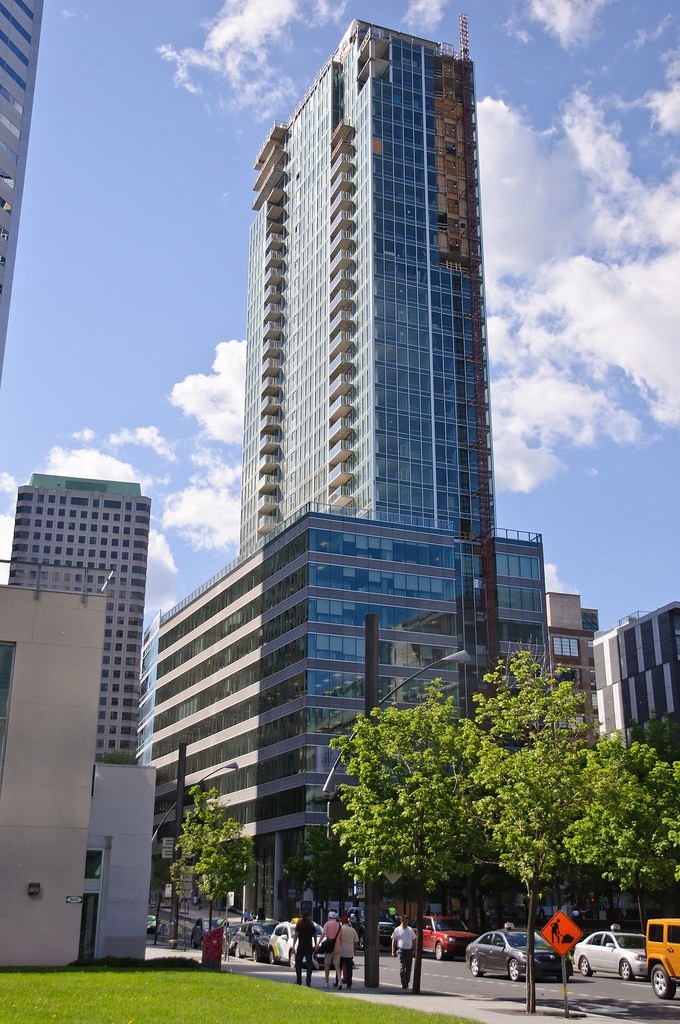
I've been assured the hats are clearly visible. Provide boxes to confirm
[329,911,339,919]
[341,916,348,923]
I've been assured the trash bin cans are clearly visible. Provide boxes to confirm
[201,926,224,971]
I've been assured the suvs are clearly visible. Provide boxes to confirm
[410,916,480,961]
[345,907,398,951]
[645,918,680,1001]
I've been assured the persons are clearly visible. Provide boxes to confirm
[391,915,417,989]
[572,907,580,919]
[242,909,254,922]
[193,895,198,909]
[537,905,545,929]
[291,911,359,990]
[426,906,433,916]
[388,905,396,922]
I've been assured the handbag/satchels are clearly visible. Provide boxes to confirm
[321,938,335,954]
[311,951,320,969]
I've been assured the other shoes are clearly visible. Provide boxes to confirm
[326,982,330,988]
[336,985,342,990]
[333,982,338,987]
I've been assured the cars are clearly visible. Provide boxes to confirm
[191,918,229,949]
[223,923,240,957]
[147,914,162,935]
[574,931,648,981]
[234,919,280,963]
[267,918,335,971]
[465,923,574,983]
